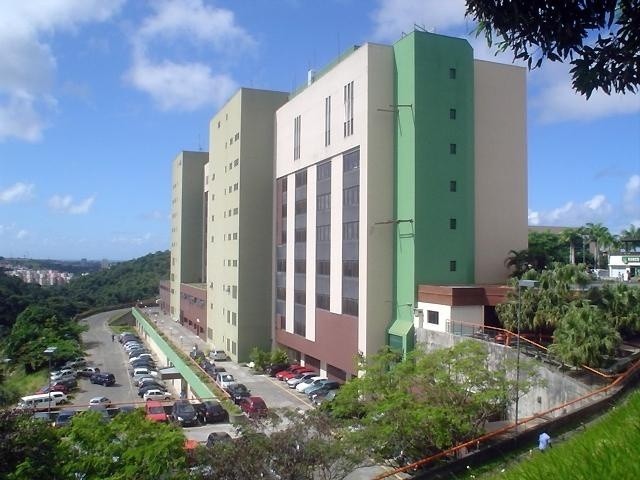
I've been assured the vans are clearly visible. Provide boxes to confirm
[589,268,605,278]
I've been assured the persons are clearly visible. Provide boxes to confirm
[537,427,552,453]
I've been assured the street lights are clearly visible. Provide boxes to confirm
[137,298,198,361]
[513,279,542,425]
